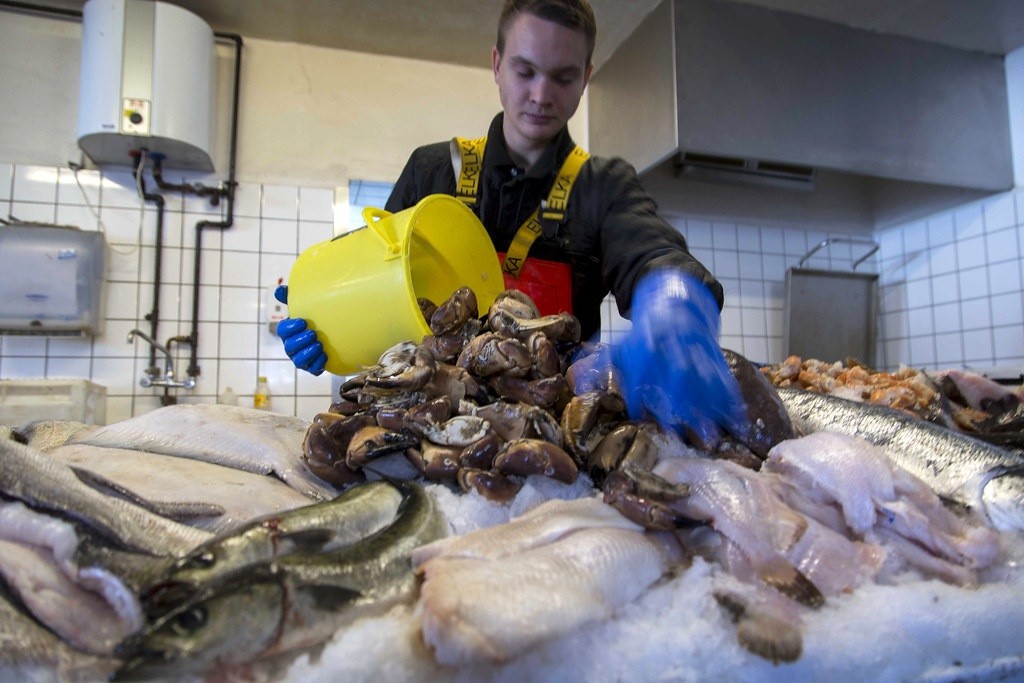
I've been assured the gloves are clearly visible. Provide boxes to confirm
[273,284,329,378]
[572,267,753,448]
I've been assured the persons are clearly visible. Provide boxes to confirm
[274,1,754,443]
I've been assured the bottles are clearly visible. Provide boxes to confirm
[218,387,238,406]
[254,377,271,412]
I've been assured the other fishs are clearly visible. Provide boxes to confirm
[0,348,1024,683]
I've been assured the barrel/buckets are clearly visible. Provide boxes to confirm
[287,193,505,376]
